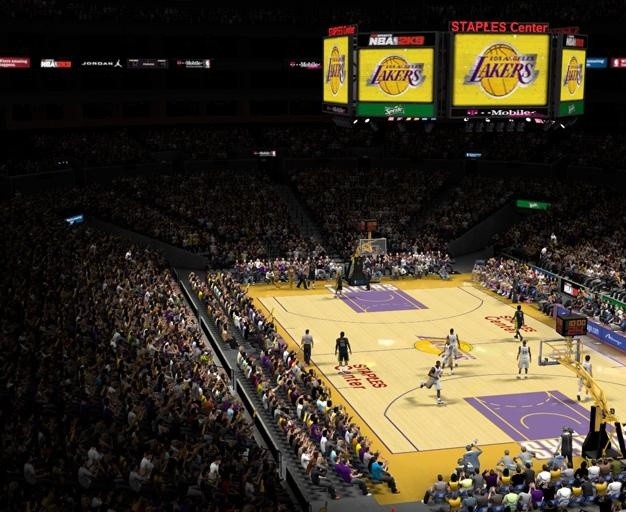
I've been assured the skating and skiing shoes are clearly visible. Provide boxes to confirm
[437,399,445,405]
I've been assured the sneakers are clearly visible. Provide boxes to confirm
[440,361,458,376]
[394,491,400,493]
[335,496,340,499]
[420,381,424,388]
[366,492,372,496]
[421,499,428,505]
[577,392,582,401]
